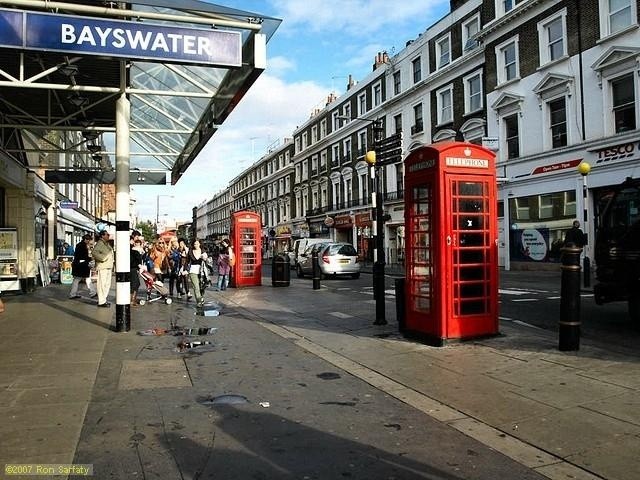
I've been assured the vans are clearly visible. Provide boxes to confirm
[283,238,333,268]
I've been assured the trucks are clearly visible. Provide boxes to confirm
[591,174,638,325]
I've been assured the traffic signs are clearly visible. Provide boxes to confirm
[376,156,402,168]
[376,141,402,152]
[376,148,402,160]
[375,133,402,146]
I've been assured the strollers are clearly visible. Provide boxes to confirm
[136,264,174,306]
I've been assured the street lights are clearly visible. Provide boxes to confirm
[333,113,390,328]
[159,213,169,217]
[155,193,176,224]
[575,160,592,288]
[363,150,378,301]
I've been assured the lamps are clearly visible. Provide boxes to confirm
[56,60,108,163]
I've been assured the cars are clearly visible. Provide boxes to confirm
[294,242,362,280]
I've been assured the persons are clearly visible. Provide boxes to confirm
[281,241,289,253]
[565,221,584,251]
[61,231,234,307]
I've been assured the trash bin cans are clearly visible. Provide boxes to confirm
[272,253,291,288]
